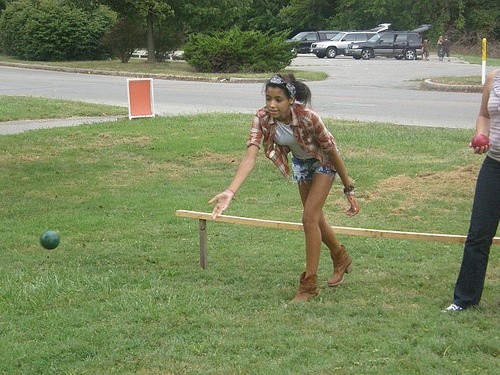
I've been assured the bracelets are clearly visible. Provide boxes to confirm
[345,191,355,195]
[226,188,235,196]
[344,185,354,191]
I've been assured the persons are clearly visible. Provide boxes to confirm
[436,35,450,62]
[422,39,430,61]
[208,73,360,303]
[441,66,500,311]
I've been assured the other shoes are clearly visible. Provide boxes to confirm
[442,305,462,314]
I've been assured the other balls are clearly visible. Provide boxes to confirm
[40,229,60,249]
[471,133,490,152]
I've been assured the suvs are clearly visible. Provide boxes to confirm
[289,31,340,54]
[310,23,392,58]
[345,24,432,60]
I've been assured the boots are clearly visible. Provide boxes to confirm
[327,246,354,286]
[289,272,318,305]
